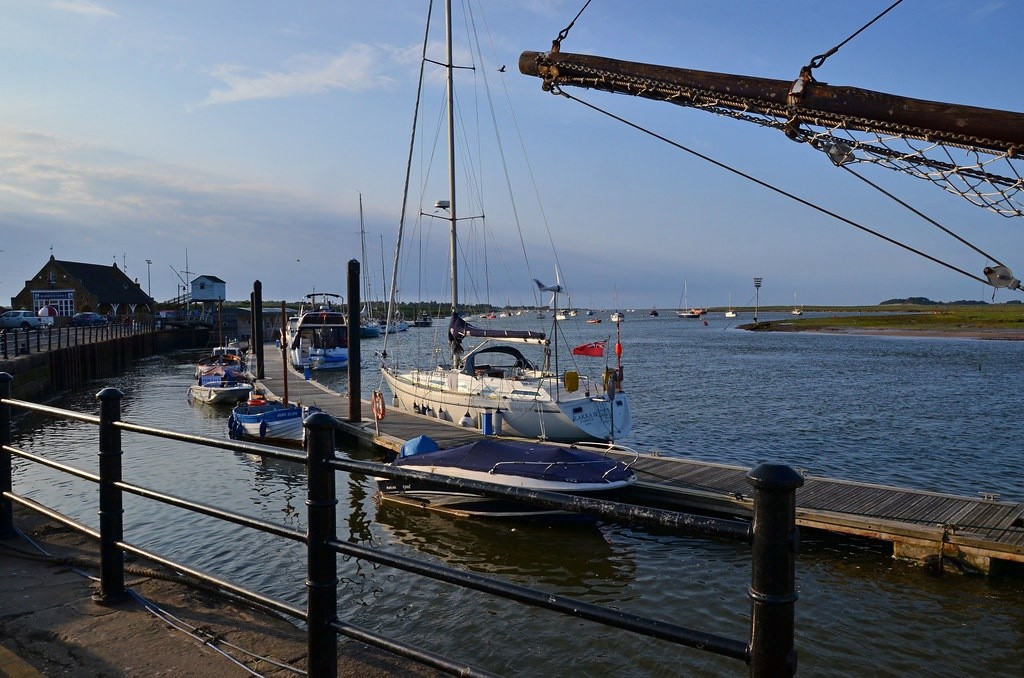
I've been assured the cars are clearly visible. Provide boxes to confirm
[70,312,106,327]
[0,310,44,331]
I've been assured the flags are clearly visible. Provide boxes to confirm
[572,340,608,356]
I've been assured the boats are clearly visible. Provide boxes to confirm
[586,309,593,316]
[194,334,249,383]
[691,308,708,316]
[535,313,546,319]
[555,310,578,322]
[227,389,307,444]
[370,434,636,498]
[185,295,253,406]
[585,317,602,324]
[279,288,435,375]
[648,305,658,316]
[462,307,530,322]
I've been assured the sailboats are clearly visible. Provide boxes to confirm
[370,1,633,447]
[725,291,737,318]
[610,282,625,322]
[792,291,803,316]
[674,280,700,319]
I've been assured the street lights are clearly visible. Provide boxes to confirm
[144,259,152,298]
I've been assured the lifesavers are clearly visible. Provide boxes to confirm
[247,399,266,406]
[371,390,386,420]
